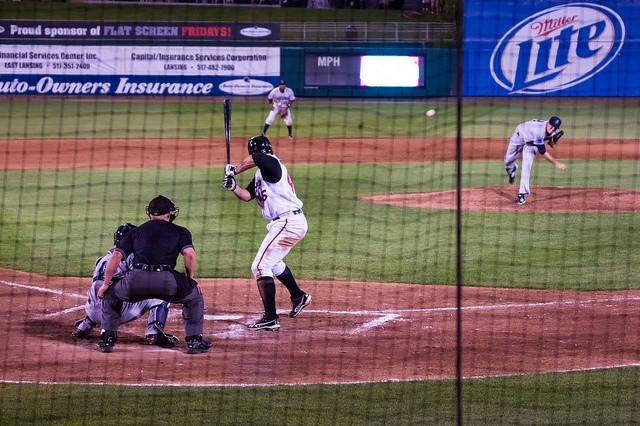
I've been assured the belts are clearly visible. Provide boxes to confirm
[134,263,171,271]
[93,276,120,282]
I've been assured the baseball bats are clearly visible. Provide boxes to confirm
[224,99,232,188]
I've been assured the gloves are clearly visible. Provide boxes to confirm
[222,175,236,191]
[224,164,237,176]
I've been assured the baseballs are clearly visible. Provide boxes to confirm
[426,110,437,118]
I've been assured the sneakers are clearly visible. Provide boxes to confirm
[185,335,212,354]
[247,315,281,332]
[146,331,180,348]
[509,163,518,184]
[95,329,117,352]
[290,290,311,318]
[289,135,294,139]
[515,194,526,205]
[72,319,93,338]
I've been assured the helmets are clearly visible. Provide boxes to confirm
[114,222,138,245]
[248,133,273,155]
[145,195,180,222]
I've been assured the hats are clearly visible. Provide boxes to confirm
[280,80,287,85]
[549,116,561,129]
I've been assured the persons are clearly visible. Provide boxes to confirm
[222,135,311,330]
[505,116,566,205]
[72,222,180,348]
[97,196,212,354]
[262,79,295,138]
